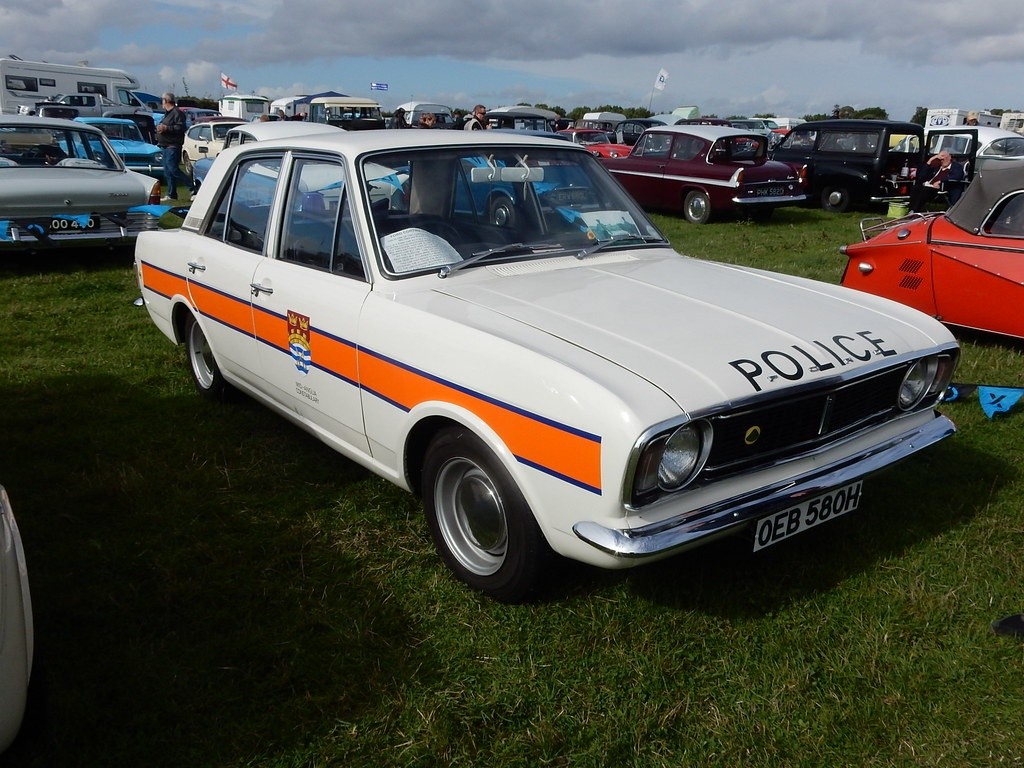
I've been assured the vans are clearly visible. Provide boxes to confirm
[0,56,154,119]
[766,118,979,215]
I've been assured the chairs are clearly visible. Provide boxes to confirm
[33,145,67,158]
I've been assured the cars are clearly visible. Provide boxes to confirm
[56,116,168,183]
[192,121,410,221]
[101,92,968,157]
[34,101,80,142]
[889,123,1024,175]
[387,144,607,233]
[593,122,807,226]
[179,121,248,176]
[0,114,162,256]
[839,156,1024,356]
[132,129,963,602]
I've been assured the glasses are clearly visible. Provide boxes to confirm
[478,112,486,115]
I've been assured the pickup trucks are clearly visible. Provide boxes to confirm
[17,93,148,121]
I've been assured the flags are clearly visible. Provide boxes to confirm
[220,72,237,92]
[654,68,669,91]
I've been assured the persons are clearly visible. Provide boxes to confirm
[389,105,493,131]
[156,92,188,201]
[256,110,289,122]
[952,111,983,153]
[905,149,964,218]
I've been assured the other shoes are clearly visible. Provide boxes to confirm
[160,196,178,201]
[190,190,197,202]
